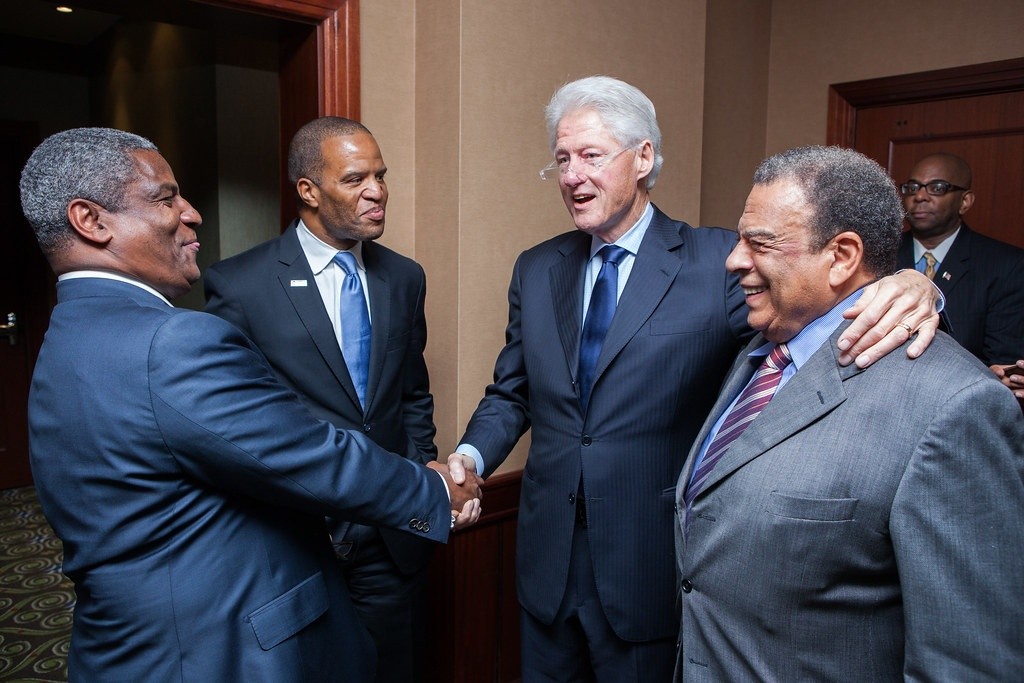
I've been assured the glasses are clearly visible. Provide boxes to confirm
[899,180,969,196]
[539,137,643,181]
[333,542,354,556]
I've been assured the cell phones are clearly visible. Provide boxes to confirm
[1003,364,1023,378]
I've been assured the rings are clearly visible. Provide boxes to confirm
[897,323,913,338]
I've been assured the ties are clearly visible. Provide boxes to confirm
[332,252,371,412]
[686,343,793,537]
[922,253,938,281]
[577,245,631,416]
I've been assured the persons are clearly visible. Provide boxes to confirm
[989,360,1024,399]
[448,75,947,683]
[20,126,482,683]
[201,114,438,683]
[674,145,1024,682]
[900,152,1024,366]
